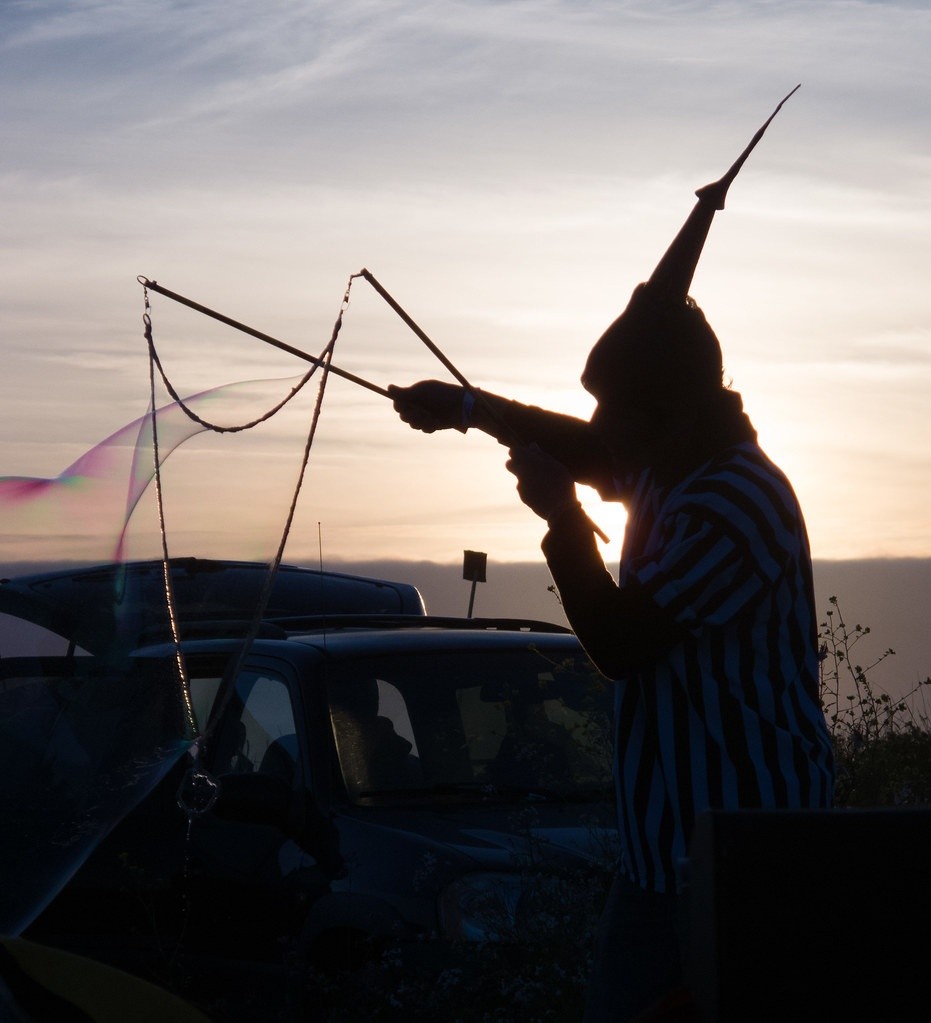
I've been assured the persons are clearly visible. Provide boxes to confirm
[387,296,843,1022]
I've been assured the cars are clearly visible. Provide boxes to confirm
[2,562,636,1023]
